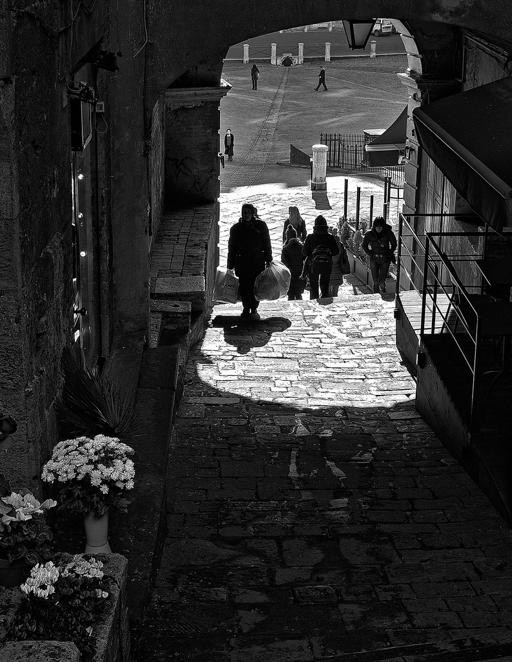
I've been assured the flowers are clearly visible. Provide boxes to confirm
[0,434,135,662]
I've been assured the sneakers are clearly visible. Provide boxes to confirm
[250,311,260,321]
[241,308,251,318]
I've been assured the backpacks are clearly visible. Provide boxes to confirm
[312,245,334,274]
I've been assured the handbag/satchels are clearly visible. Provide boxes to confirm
[254,261,292,303]
[216,268,239,302]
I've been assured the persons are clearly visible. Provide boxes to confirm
[250,64,259,90]
[226,203,272,319]
[327,223,345,298]
[283,205,307,244]
[224,128,234,160]
[301,214,340,300]
[281,224,305,300]
[361,216,398,293]
[313,65,328,91]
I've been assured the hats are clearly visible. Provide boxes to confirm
[313,215,329,229]
[285,224,297,239]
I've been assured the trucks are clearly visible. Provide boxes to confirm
[371,19,400,37]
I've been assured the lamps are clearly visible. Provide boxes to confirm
[342,17,378,51]
